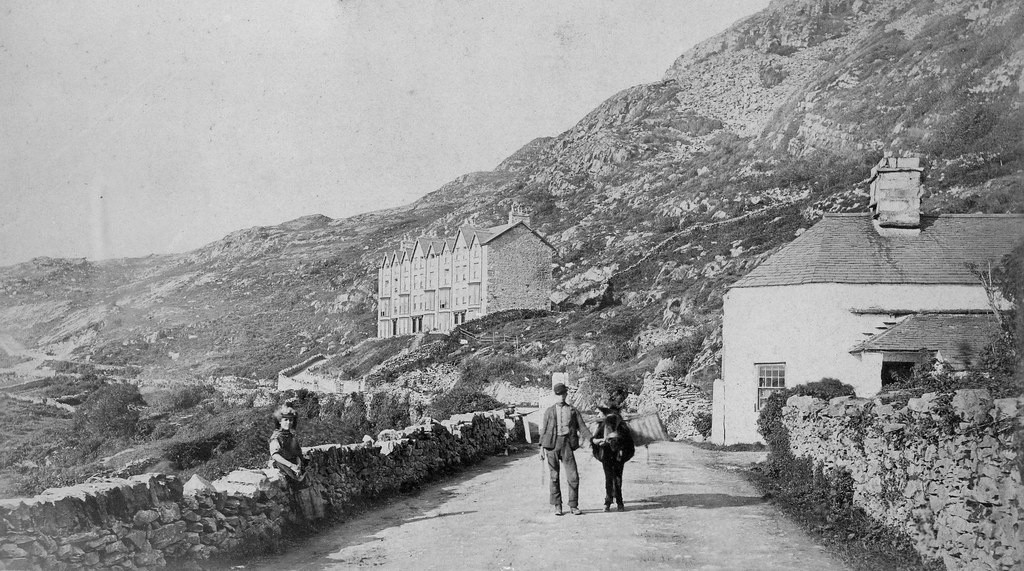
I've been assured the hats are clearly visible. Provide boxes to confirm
[554,383,569,394]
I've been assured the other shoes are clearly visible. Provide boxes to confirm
[555,504,562,515]
[570,506,581,515]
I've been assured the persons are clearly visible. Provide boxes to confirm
[538,383,603,515]
[269,408,322,533]
